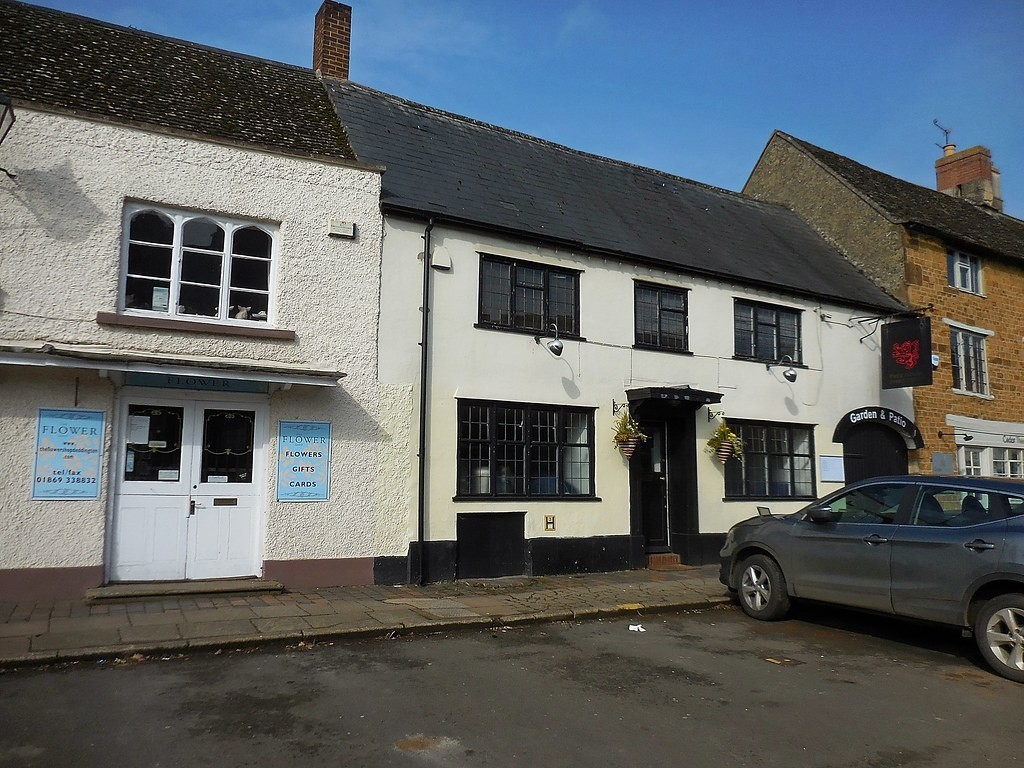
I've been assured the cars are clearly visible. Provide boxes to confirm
[719,474,1024,688]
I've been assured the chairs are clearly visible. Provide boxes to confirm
[918,494,943,518]
[962,495,987,515]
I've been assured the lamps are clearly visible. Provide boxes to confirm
[534,323,563,356]
[0,97,17,178]
[766,355,797,382]
